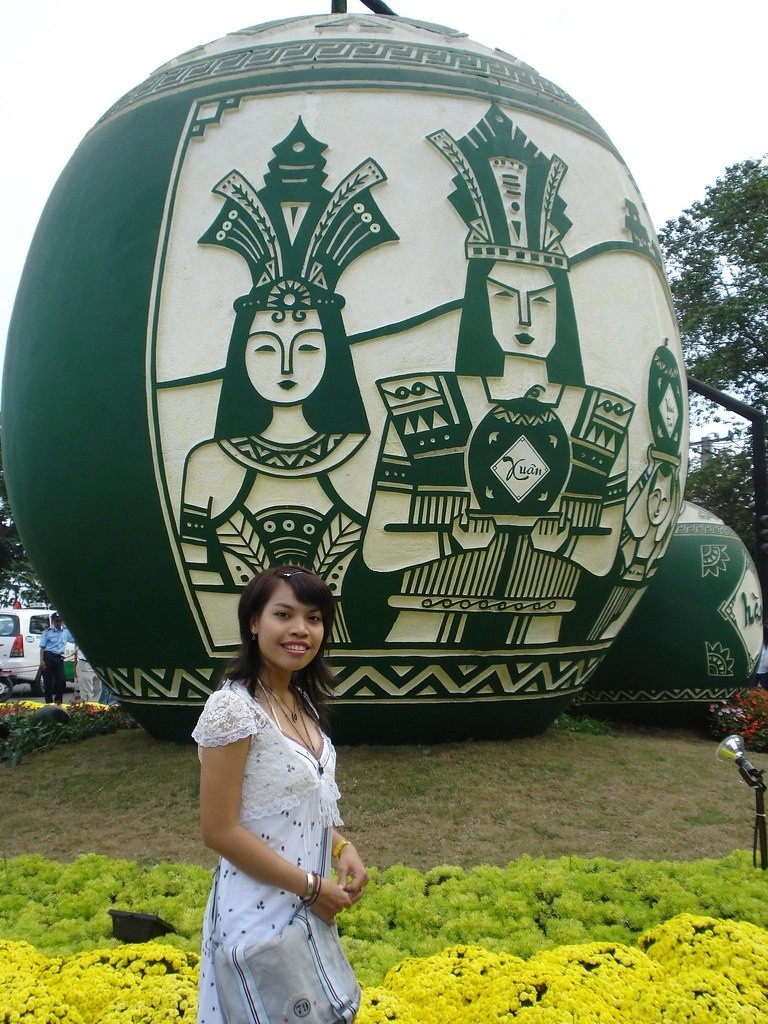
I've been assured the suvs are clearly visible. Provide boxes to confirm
[0,603,78,704]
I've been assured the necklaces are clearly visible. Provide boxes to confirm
[262,682,324,775]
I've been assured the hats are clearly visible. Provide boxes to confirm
[51,612,62,623]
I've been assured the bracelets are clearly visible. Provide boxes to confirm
[333,839,353,861]
[288,872,321,939]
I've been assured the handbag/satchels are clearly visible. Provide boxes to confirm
[210,901,361,1024]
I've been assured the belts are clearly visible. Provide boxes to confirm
[79,659,88,662]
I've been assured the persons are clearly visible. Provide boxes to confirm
[191,564,370,1024]
[38,612,76,705]
[76,646,102,702]
